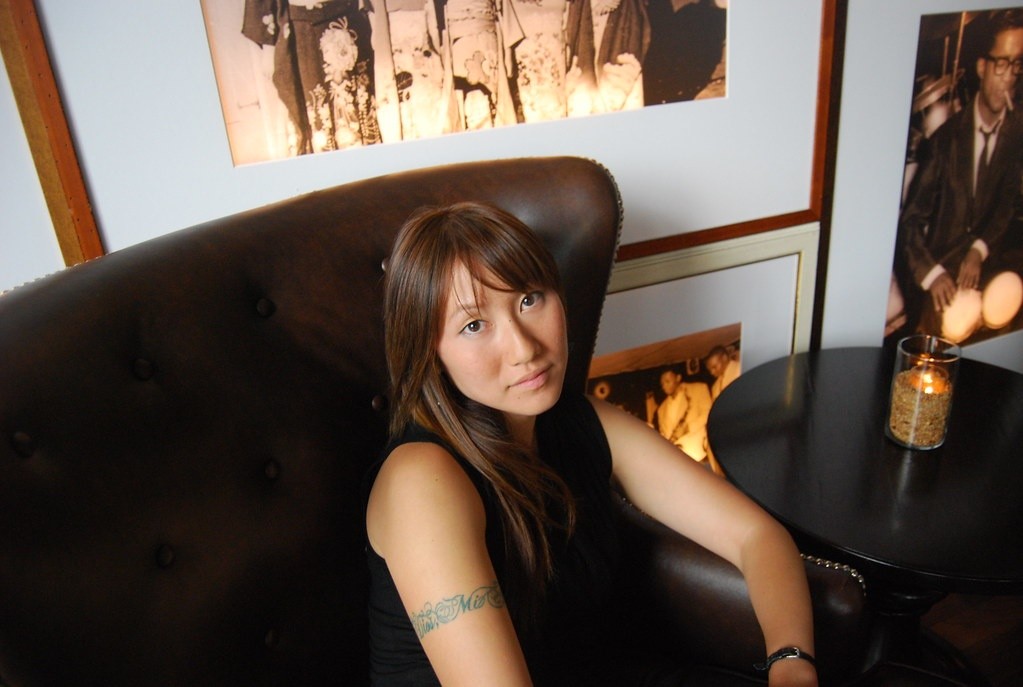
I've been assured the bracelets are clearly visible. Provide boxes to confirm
[754,647,817,669]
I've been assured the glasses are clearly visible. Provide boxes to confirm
[984,53,1023,75]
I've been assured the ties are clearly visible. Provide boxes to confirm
[975,120,1000,194]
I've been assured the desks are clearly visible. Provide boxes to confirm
[705,346,1023,686]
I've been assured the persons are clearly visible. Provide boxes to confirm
[656,345,738,473]
[362,202,819,687]
[899,12,1023,310]
[240,0,725,152]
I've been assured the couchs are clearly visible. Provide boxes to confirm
[0,156,888,687]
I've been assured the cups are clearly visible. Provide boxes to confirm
[885,335,961,451]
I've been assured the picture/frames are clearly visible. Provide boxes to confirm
[1,0,847,463]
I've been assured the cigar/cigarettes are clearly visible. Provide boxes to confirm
[1004,91,1013,113]
